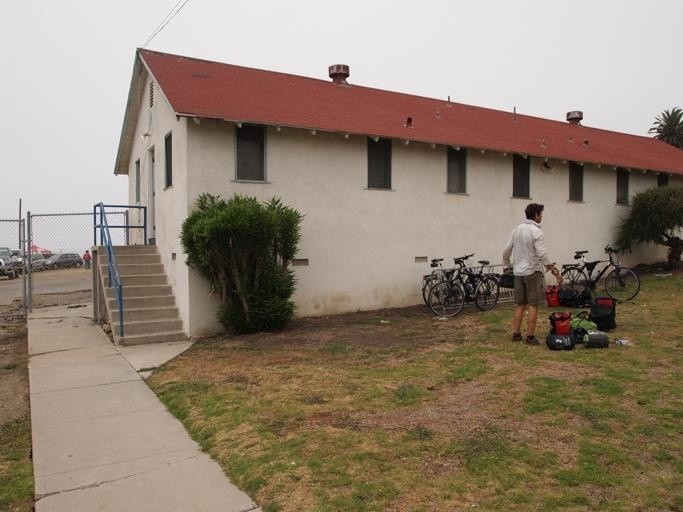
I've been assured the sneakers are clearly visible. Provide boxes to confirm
[512,334,545,346]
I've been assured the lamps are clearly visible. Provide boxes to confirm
[140,132,150,140]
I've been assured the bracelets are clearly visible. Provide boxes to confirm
[551,267,559,275]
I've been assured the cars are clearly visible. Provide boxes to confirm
[0,245,84,281]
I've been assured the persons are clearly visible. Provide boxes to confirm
[498,202,564,345]
[82,249,91,271]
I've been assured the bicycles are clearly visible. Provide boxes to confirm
[420,241,640,320]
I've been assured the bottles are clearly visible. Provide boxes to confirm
[591,269,600,281]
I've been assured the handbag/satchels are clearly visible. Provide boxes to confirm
[497,274,514,288]
[546,297,616,351]
[545,286,592,308]
